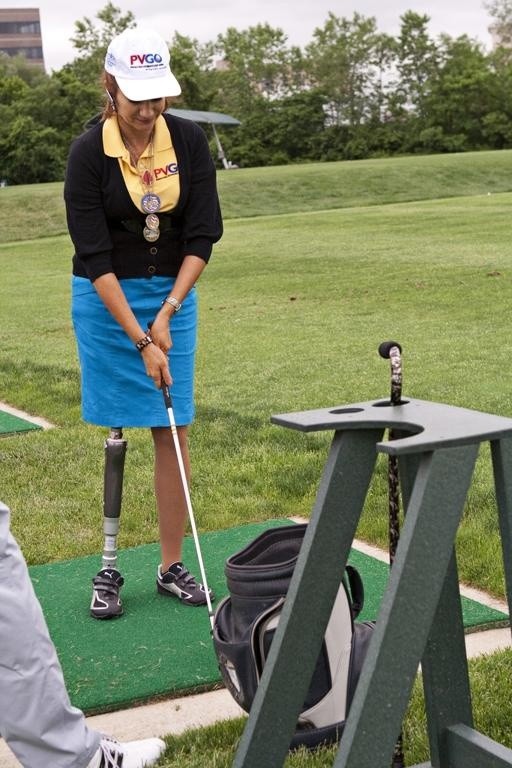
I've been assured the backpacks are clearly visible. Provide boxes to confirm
[209,522,380,754]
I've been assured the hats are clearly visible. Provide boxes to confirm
[104,26,183,102]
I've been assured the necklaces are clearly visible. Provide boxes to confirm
[121,132,162,242]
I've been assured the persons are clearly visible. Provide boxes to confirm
[59,28,227,623]
[0,498,171,766]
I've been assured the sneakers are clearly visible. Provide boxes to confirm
[155,561,216,607]
[90,568,125,620]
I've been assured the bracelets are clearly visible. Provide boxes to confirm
[161,295,184,313]
[137,335,152,351]
